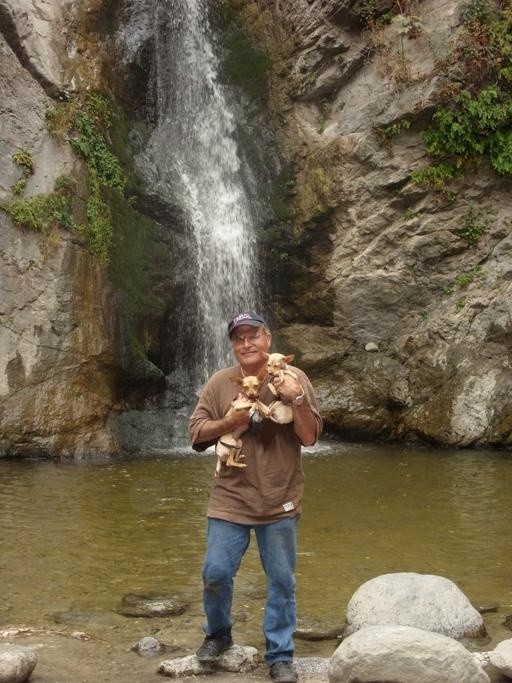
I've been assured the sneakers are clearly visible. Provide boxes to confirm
[196,634,233,661]
[269,659,299,683]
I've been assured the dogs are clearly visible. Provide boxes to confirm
[214,368,267,480]
[254,349,297,427]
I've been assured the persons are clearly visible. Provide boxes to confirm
[188,312,323,683]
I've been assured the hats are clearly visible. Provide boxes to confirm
[228,311,264,338]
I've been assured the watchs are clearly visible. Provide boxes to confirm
[292,384,306,407]
